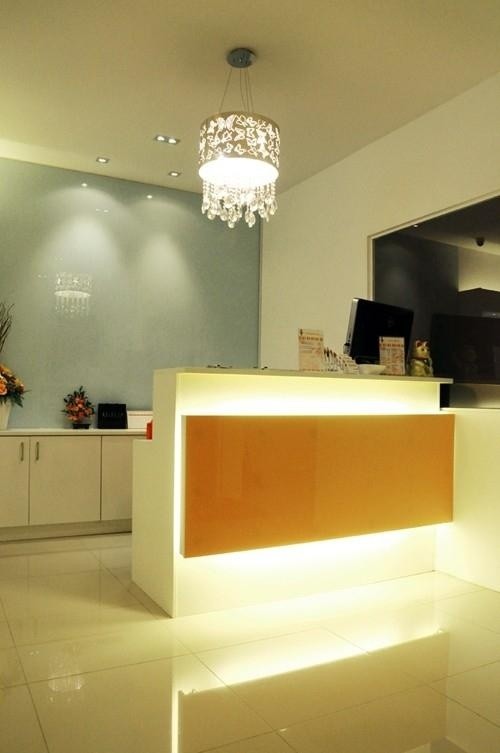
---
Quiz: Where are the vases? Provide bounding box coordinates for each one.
[72,422,92,428]
[0,398,13,430]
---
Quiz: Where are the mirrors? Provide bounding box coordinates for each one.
[365,191,500,409]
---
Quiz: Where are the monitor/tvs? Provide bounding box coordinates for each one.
[342,297,415,370]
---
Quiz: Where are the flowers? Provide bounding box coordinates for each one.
[0,301,26,408]
[60,385,98,423]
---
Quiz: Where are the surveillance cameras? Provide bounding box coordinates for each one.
[474,236,486,247]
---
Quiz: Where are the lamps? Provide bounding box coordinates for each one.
[53,270,94,318]
[197,47,282,230]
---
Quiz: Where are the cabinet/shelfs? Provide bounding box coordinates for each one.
[0,398,151,543]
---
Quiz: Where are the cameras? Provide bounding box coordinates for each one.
[431,311,499,384]
